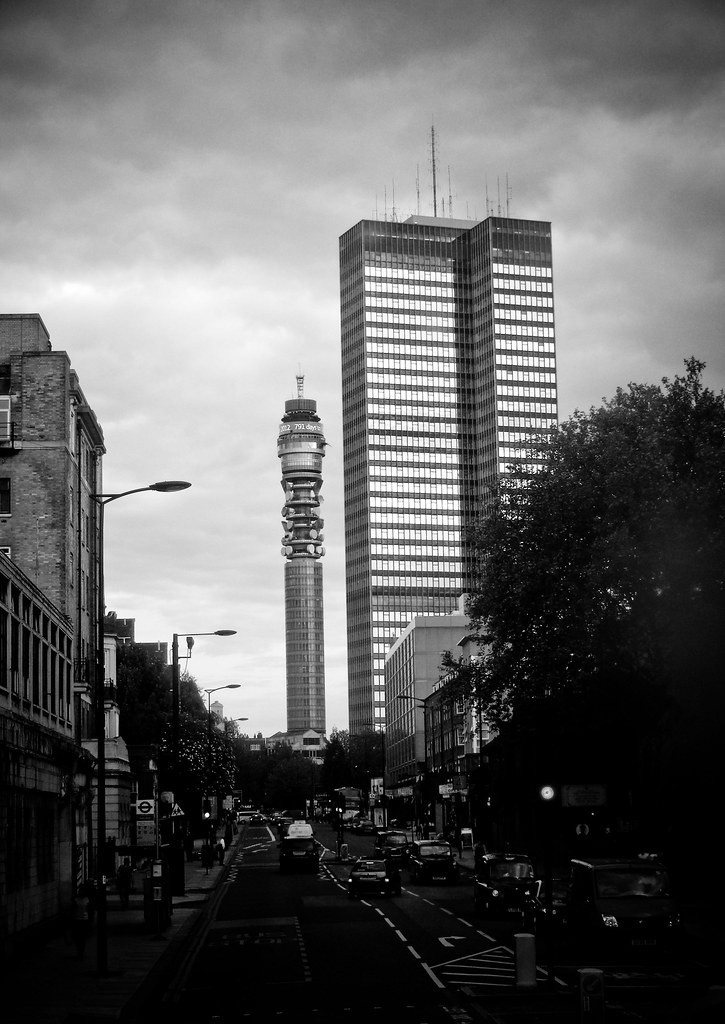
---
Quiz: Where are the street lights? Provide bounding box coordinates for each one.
[442,660,483,765]
[362,722,388,831]
[169,629,237,797]
[416,704,436,786]
[223,717,249,738]
[397,696,429,781]
[203,683,241,798]
[91,479,191,971]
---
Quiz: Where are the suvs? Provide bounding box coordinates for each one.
[519,854,687,965]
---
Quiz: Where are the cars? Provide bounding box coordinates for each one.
[406,837,458,886]
[336,853,404,900]
[237,806,375,849]
[469,849,540,923]
[372,828,411,865]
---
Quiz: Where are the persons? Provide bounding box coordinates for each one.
[114,858,135,902]
[455,836,466,859]
[222,808,237,833]
[473,839,487,871]
[311,802,332,824]
[413,823,430,840]
